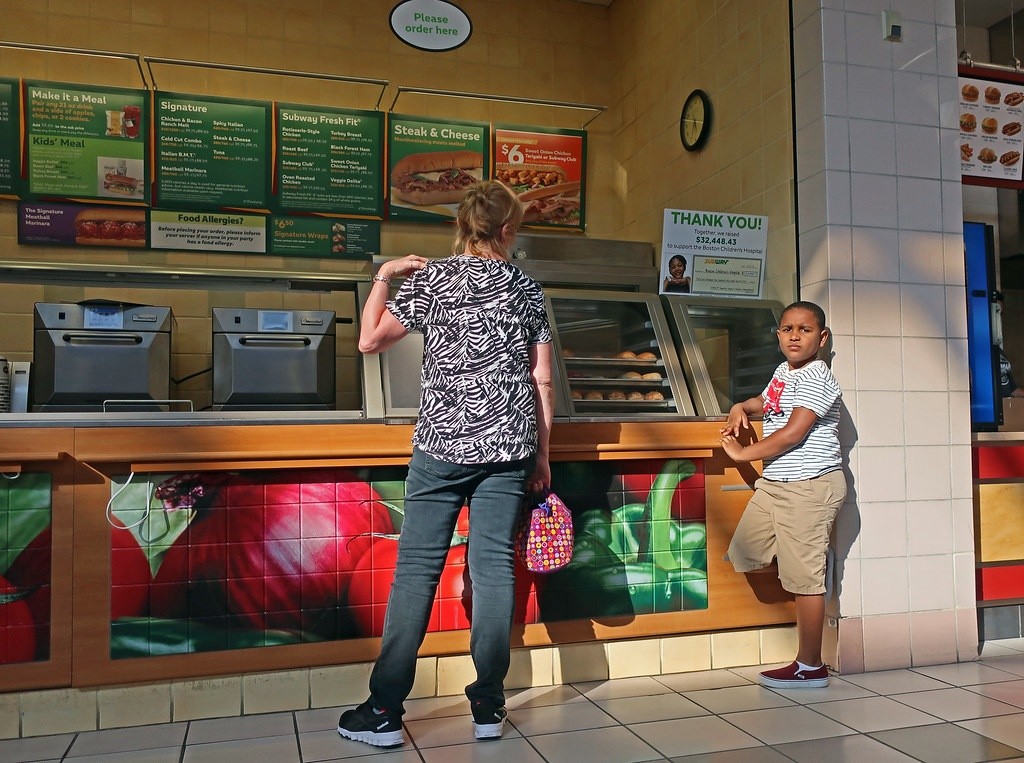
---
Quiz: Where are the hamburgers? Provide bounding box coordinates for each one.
[977,148,997,164]
[959,84,1001,133]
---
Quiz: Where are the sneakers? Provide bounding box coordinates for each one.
[759,660,829,689]
[472,702,508,739]
[824,547,834,601]
[337,700,405,746]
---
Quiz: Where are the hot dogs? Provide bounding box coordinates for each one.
[496,163,567,195]
[1004,92,1024,106]
[109,176,138,195]
[391,152,483,205]
[1002,122,1022,136]
[518,181,581,224]
[75,209,145,247]
[999,151,1021,166]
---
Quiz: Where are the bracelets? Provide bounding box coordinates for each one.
[373,275,391,285]
[665,276,672,282]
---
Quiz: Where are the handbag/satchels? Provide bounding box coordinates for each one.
[515,482,575,573]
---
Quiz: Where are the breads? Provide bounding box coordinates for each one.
[613,351,657,360]
[563,349,574,357]
[572,390,664,400]
[618,372,661,379]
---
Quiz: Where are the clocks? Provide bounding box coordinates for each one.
[679,89,712,151]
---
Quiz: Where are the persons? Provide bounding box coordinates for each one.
[718,301,847,688]
[336,180,554,747]
[662,255,691,293]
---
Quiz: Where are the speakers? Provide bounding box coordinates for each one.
[10,361,32,413]
